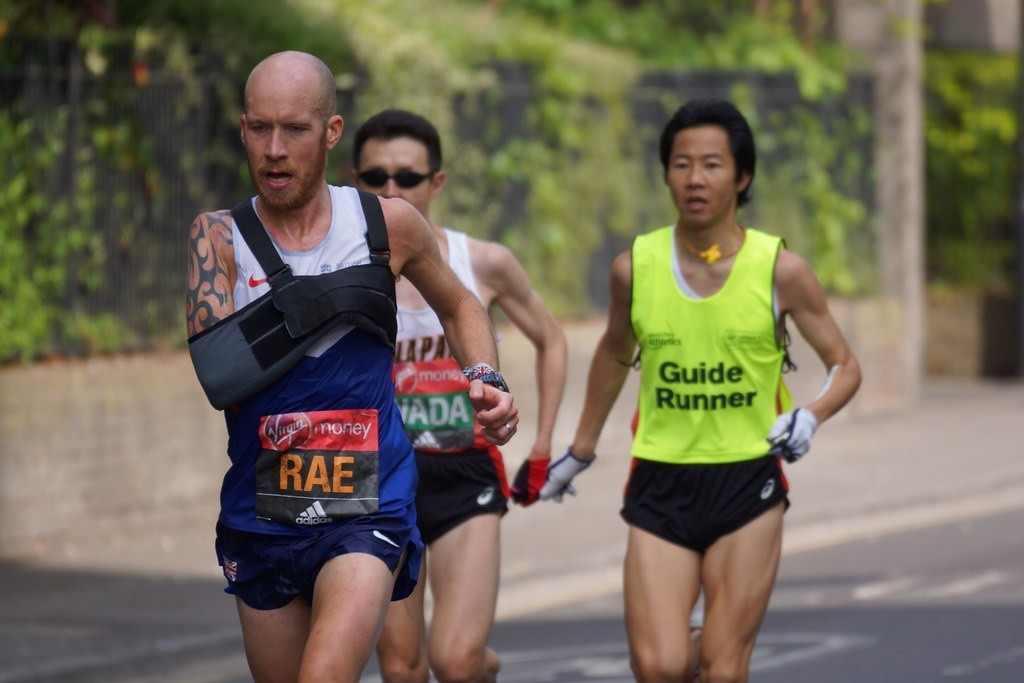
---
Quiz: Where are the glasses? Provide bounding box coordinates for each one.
[358,169,432,189]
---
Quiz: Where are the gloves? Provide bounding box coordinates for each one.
[538,447,597,503]
[509,458,550,507]
[765,407,818,464]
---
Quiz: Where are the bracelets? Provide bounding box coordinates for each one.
[463,363,493,379]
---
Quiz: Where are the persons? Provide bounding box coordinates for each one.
[186,50,520,683]
[539,99,861,683]
[352,109,568,682]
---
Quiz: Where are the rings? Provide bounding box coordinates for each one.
[504,423,512,432]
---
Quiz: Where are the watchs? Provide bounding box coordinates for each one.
[479,373,509,392]
